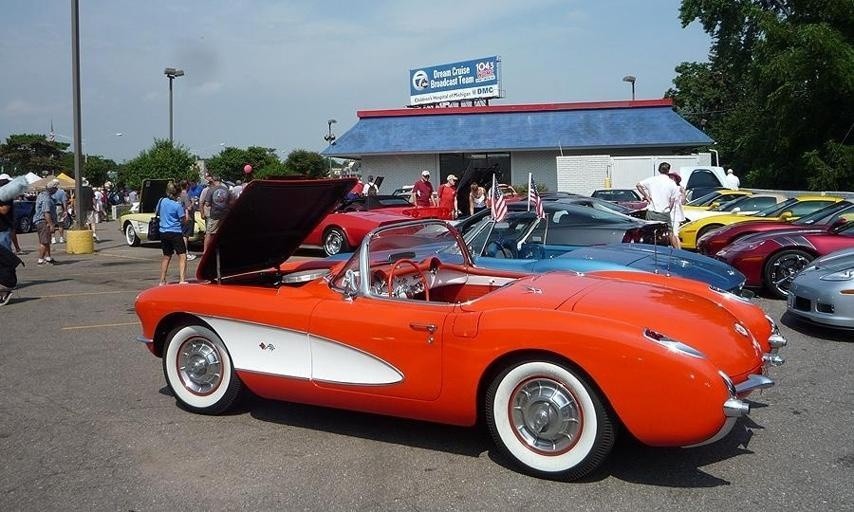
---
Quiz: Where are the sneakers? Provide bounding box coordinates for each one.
[37,258,53,267]
[51,237,56,244]
[179,282,189,284]
[93,233,96,241]
[0,292,14,306]
[15,250,27,254]
[46,256,60,265]
[159,281,167,287]
[59,237,64,243]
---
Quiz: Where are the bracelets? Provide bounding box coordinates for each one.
[454,210,458,212]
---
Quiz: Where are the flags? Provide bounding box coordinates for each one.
[487,178,507,222]
[530,174,545,221]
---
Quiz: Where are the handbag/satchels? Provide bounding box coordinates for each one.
[408,193,416,204]
[148,218,161,241]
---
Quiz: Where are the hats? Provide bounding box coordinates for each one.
[367,176,373,180]
[668,171,682,183]
[0,173,13,180]
[422,171,430,176]
[46,181,63,189]
[203,172,221,182]
[447,174,458,180]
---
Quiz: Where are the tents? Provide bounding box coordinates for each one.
[22,173,75,198]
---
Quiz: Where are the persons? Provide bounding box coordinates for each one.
[468,182,486,216]
[33,179,66,265]
[0,245,22,307]
[155,173,249,288]
[722,169,740,190]
[635,161,678,249]
[66,188,75,215]
[411,171,437,208]
[0,173,13,253]
[668,171,687,250]
[437,174,459,221]
[81,177,137,243]
[350,174,379,197]
[8,229,25,255]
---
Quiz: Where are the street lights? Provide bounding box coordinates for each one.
[164,67,184,152]
[46,131,126,165]
[324,118,337,176]
[623,74,636,100]
[170,142,227,161]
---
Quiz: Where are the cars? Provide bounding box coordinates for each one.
[11,199,72,232]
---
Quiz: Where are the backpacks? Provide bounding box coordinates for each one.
[368,183,376,198]
[210,186,230,220]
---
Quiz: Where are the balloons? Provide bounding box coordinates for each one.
[243,164,252,173]
